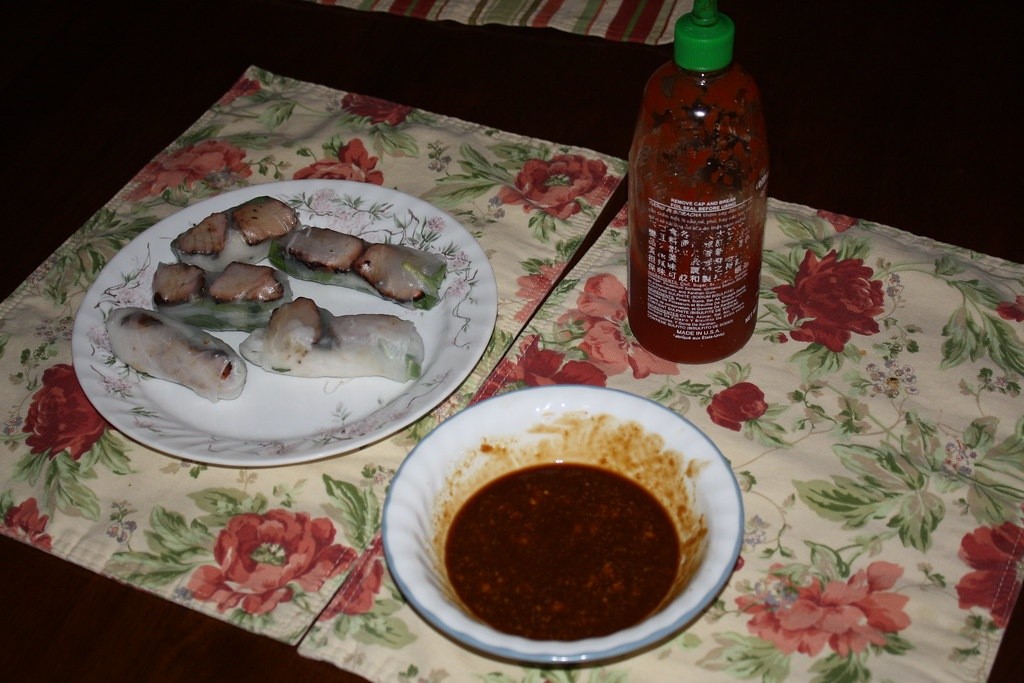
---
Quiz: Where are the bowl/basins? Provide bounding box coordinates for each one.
[381,383,744,666]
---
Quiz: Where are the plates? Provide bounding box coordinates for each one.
[71,178,499,468]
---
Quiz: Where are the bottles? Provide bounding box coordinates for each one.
[625,0,769,365]
